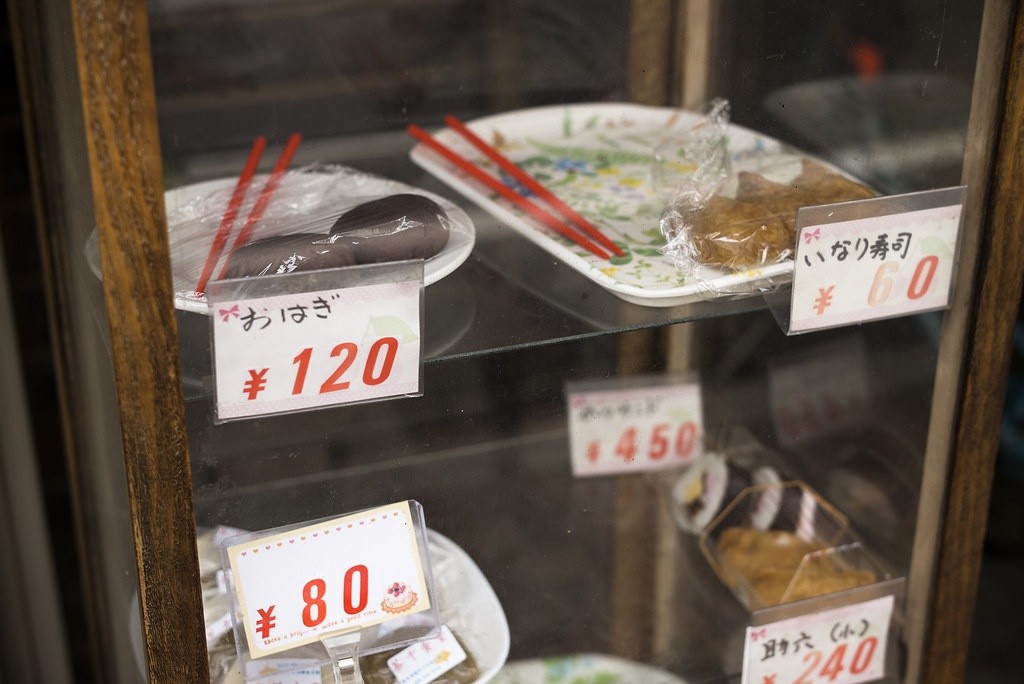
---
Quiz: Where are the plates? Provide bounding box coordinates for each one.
[131,522,511,684]
[85,172,477,315]
[488,651,689,684]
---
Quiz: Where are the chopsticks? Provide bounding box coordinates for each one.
[409,117,626,261]
[192,134,299,300]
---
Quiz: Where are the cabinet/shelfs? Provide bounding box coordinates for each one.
[71,97,945,684]
